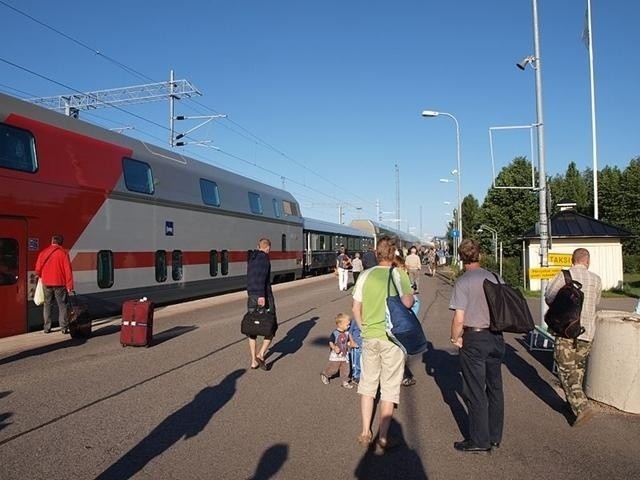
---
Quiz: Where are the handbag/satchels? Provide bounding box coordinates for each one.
[241,306,278,340]
[343,259,351,268]
[482,278,536,335]
[544,281,584,339]
[385,295,428,356]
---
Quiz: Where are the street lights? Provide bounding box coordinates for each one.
[440,178,461,266]
[418,106,463,268]
[338,205,364,226]
[476,223,498,262]
[444,200,458,251]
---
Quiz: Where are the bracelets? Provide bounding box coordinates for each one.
[450,338,457,345]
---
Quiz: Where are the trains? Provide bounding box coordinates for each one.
[0,91,447,342]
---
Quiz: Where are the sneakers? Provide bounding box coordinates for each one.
[572,406,597,428]
[402,377,417,386]
[252,353,272,371]
[320,373,360,390]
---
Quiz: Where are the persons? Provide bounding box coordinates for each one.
[448,239,507,454]
[544,249,602,426]
[35,234,76,334]
[336,246,450,294]
[348,319,362,385]
[351,236,413,456]
[392,255,420,387]
[320,313,353,389]
[246,238,278,371]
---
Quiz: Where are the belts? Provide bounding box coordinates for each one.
[462,326,491,333]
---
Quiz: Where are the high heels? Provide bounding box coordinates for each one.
[357,432,403,457]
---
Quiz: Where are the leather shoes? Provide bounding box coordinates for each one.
[454,439,500,452]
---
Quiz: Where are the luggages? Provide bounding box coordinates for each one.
[64,290,92,341]
[120,297,154,348]
